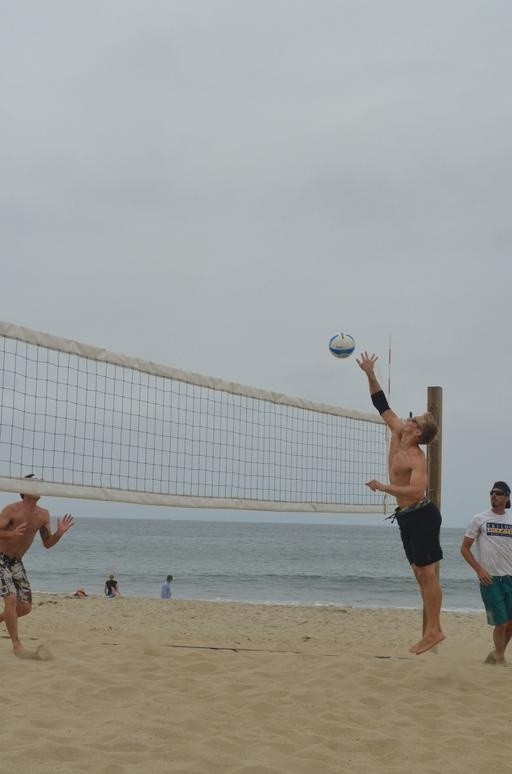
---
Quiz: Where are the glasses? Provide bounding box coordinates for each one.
[490,490,504,496]
[412,416,420,430]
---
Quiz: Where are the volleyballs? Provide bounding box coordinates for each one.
[329,333,355,358]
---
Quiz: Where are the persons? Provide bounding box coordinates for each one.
[161,575,173,599]
[0,474,74,654]
[356,350,445,656]
[104,575,118,598]
[461,480,512,662]
[75,586,88,600]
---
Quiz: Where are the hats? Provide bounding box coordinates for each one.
[493,481,510,508]
